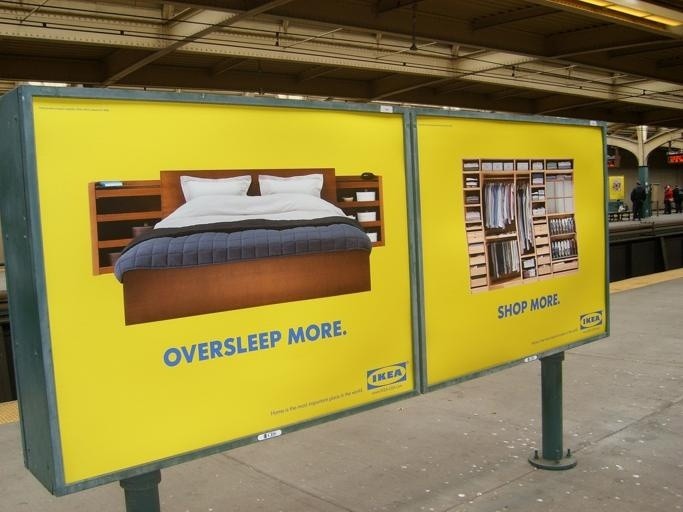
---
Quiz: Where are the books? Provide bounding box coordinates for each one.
[462,157,577,278]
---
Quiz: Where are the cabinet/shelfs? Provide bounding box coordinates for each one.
[335,174,385,247]
[461,157,580,292]
[86,178,163,275]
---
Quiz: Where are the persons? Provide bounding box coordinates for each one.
[617,199,625,212]
[664,184,673,214]
[630,181,647,221]
[672,184,682,213]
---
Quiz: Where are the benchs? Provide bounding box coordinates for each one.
[608,210,631,221]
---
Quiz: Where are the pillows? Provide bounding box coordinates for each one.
[257,173,324,198]
[179,175,253,202]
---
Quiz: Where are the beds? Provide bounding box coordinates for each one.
[114,168,373,325]
